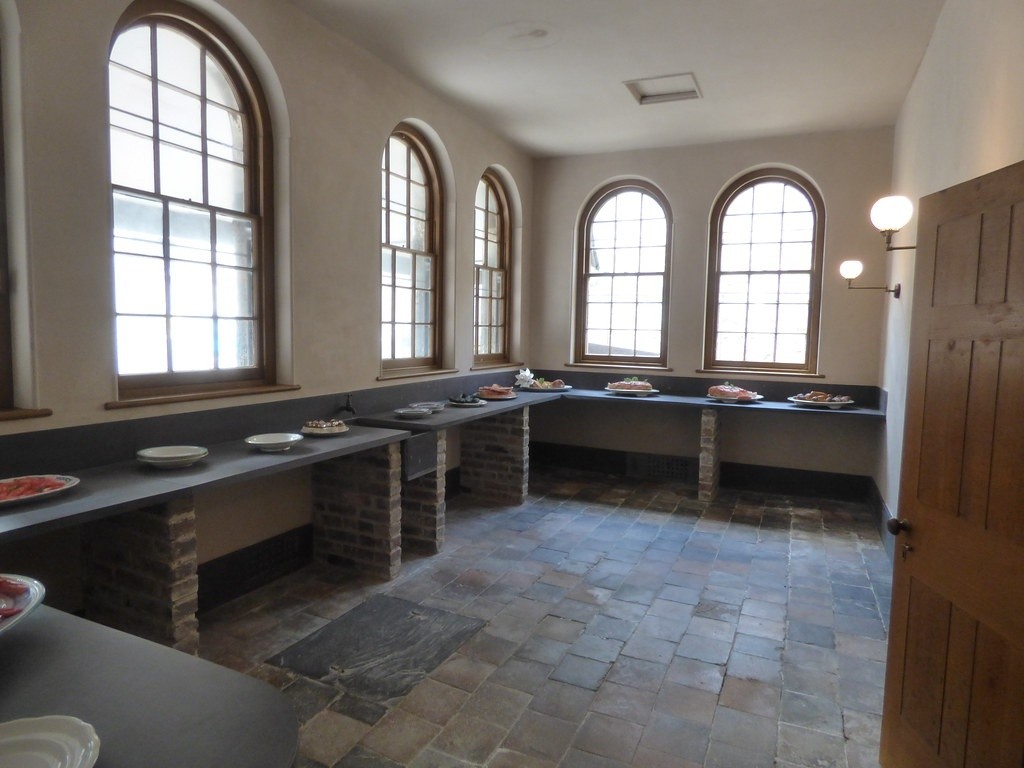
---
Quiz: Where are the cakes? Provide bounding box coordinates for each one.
[301,418,349,433]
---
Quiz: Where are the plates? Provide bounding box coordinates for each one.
[706,394,764,403]
[0,715,100,768]
[300,427,353,437]
[136,446,209,469]
[519,385,572,392]
[393,408,433,419]
[451,399,487,408]
[472,392,520,399]
[604,387,660,397]
[0,474,80,507]
[408,401,446,414]
[0,573,45,635]
[244,433,305,452]
[787,396,855,409]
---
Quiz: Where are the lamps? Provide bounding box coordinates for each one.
[870,195,916,251]
[840,260,900,298]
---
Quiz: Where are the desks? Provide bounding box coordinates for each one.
[0,386,885,768]
